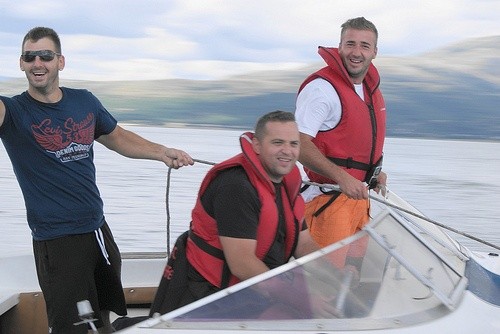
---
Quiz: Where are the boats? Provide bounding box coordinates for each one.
[0,179,500,333]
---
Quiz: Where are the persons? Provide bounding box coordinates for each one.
[0,25,194,334]
[147,109,361,323]
[292,15,388,319]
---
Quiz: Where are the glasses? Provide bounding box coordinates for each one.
[21,49,61,62]
